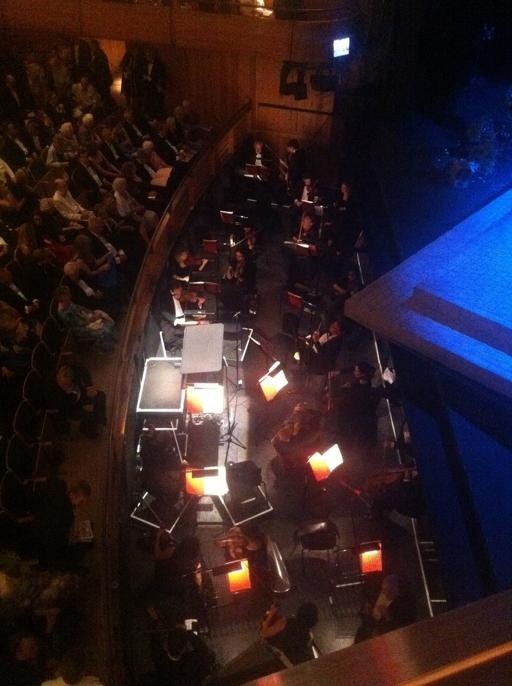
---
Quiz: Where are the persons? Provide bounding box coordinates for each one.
[0,35,412,686]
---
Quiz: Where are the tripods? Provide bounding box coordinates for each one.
[227,319,256,403]
[219,367,248,449]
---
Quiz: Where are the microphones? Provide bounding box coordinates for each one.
[223,356,228,367]
[233,312,240,318]
